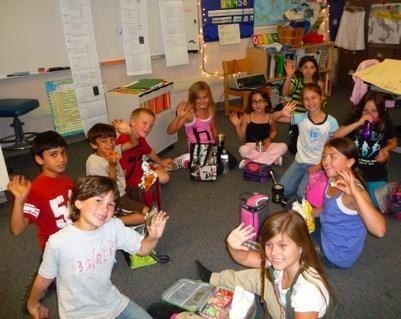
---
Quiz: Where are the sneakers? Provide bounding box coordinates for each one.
[164,162,179,172]
[239,159,246,169]
[274,156,283,166]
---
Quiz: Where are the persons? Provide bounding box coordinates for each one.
[228,89,290,170]
[334,92,398,213]
[86,118,156,222]
[30,176,170,318]
[279,55,331,157]
[9,130,151,311]
[268,83,342,199]
[146,213,338,318]
[281,134,389,273]
[118,107,191,206]
[167,81,239,175]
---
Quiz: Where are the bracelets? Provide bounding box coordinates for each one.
[266,135,273,142]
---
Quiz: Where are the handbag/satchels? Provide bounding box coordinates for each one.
[189,143,218,181]
[129,203,169,270]
[240,191,271,241]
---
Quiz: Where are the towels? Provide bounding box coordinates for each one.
[335,10,366,51]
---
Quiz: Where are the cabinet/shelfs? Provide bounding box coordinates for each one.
[104,79,178,163]
[247,31,335,96]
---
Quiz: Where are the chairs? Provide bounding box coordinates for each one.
[223,58,271,118]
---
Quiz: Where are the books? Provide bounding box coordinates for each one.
[114,78,165,92]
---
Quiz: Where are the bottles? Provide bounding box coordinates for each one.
[221,148,231,175]
[361,114,372,139]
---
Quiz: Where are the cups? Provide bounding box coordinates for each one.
[255,140,266,152]
[271,184,284,202]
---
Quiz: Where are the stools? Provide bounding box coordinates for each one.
[0,100,42,174]
